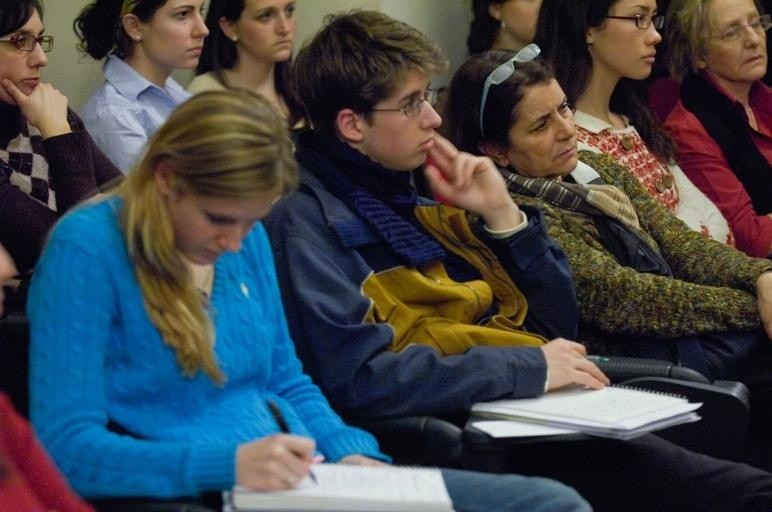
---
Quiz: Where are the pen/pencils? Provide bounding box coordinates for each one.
[266,399,316,482]
[587,355,609,363]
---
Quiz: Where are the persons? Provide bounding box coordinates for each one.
[1,2,772,512]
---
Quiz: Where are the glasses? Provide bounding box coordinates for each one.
[372,89,438,118]
[721,14,772,42]
[480,43,541,137]
[0,33,53,52]
[602,14,664,29]
